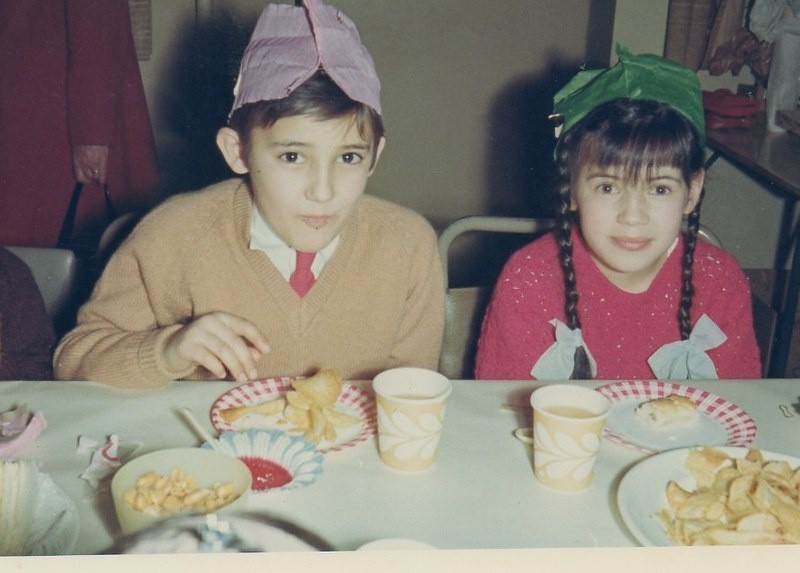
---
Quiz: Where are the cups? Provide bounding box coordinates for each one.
[530,383,612,488]
[108,448,253,536]
[371,366,452,472]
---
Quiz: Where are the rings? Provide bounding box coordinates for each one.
[93,168,99,173]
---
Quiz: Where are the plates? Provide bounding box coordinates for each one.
[596,380,756,453]
[211,375,378,455]
[616,446,800,545]
[0,475,78,556]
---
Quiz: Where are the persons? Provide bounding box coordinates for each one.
[48,0,445,379]
[0,0,161,381]
[478,44,761,379]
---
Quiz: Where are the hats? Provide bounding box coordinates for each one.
[227,1,382,126]
[553,43,706,153]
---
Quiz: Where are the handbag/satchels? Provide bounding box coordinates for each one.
[48,177,117,338]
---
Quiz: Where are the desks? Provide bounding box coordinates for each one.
[703,111,800,379]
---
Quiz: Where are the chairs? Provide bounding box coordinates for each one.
[437,215,724,380]
[6,245,78,331]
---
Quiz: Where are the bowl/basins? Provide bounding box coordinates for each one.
[201,430,324,495]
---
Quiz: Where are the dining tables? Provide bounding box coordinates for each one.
[0,378,800,556]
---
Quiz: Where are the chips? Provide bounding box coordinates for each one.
[285,366,341,405]
[655,445,800,546]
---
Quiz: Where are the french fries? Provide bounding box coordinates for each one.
[220,398,358,442]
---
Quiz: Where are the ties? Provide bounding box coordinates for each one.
[289,251,316,297]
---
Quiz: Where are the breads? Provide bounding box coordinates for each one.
[637,396,697,425]
